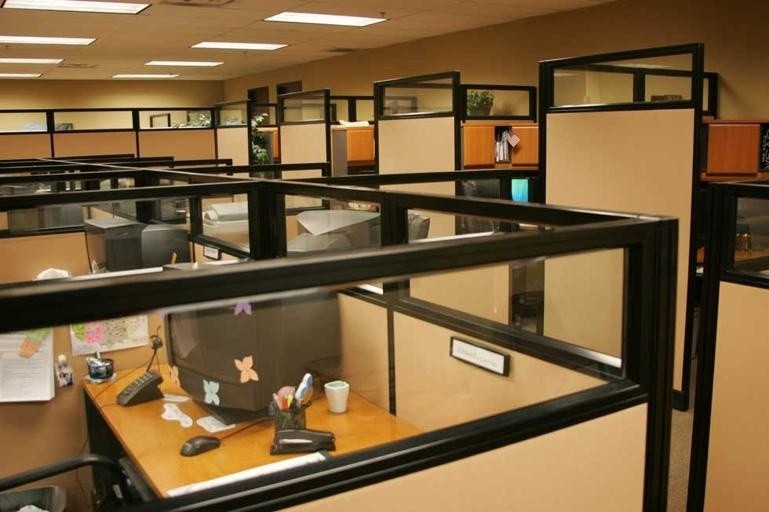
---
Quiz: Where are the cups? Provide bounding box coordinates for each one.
[323,380,350,414]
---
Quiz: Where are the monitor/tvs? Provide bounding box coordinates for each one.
[84,217,190,275]
[8,204,83,230]
[163,262,341,424]
[510,176,531,203]
[287,210,381,254]
[205,201,249,225]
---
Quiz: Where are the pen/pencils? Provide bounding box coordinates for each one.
[271,384,314,430]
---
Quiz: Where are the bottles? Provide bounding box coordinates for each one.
[741,234,752,257]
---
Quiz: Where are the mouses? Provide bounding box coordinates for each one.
[180,437,220,455]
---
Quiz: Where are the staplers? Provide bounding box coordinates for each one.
[269,429,336,454]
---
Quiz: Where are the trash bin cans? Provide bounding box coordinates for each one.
[512,289,543,316]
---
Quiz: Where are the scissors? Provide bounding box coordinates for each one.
[295,373,315,406]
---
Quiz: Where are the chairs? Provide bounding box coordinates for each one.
[0,454,130,512]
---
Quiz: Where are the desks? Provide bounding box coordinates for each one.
[80,359,424,512]
